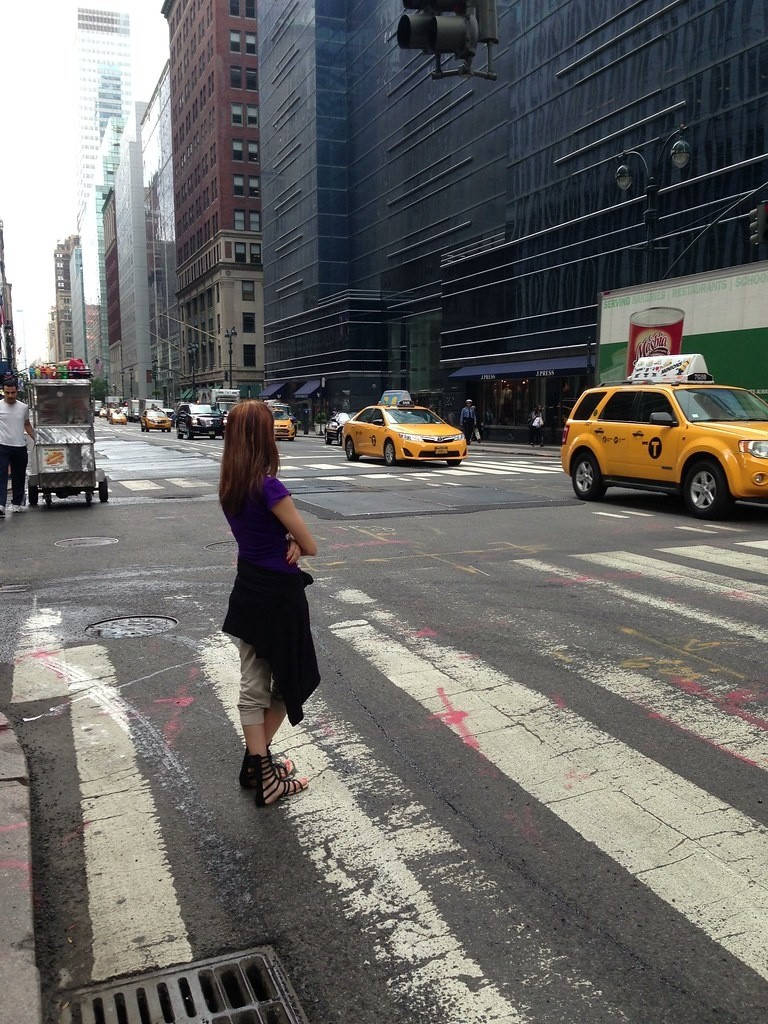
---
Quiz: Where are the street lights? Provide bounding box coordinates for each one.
[613,124,695,284]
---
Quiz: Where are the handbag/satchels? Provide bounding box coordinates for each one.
[531,413,544,428]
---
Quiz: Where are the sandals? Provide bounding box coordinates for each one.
[239,738,294,791]
[248,748,309,808]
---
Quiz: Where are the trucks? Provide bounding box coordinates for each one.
[138,399,176,425]
[128,398,141,423]
[197,389,241,434]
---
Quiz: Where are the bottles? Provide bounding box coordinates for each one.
[30,365,90,379]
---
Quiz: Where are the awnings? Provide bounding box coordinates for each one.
[292,380,325,399]
[181,385,222,402]
[447,355,595,383]
[256,383,288,398]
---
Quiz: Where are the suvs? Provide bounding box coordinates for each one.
[176,401,227,440]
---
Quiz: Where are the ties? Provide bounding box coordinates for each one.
[467,408,471,420]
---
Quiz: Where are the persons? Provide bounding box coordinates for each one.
[470,405,482,443]
[527,402,544,447]
[460,399,476,444]
[502,384,513,422]
[0,378,35,517]
[217,399,322,808]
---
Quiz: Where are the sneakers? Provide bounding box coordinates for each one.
[12,504,22,513]
[0,505,7,515]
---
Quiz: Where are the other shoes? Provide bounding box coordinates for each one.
[478,439,480,443]
[466,441,469,445]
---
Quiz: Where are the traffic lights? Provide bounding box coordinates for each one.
[748,200,768,246]
[396,1,499,81]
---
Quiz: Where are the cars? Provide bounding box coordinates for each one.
[342,389,469,467]
[324,412,359,446]
[265,406,296,442]
[560,354,768,520]
[95,400,128,425]
[140,404,172,432]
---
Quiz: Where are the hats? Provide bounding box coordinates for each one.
[465,399,472,403]
